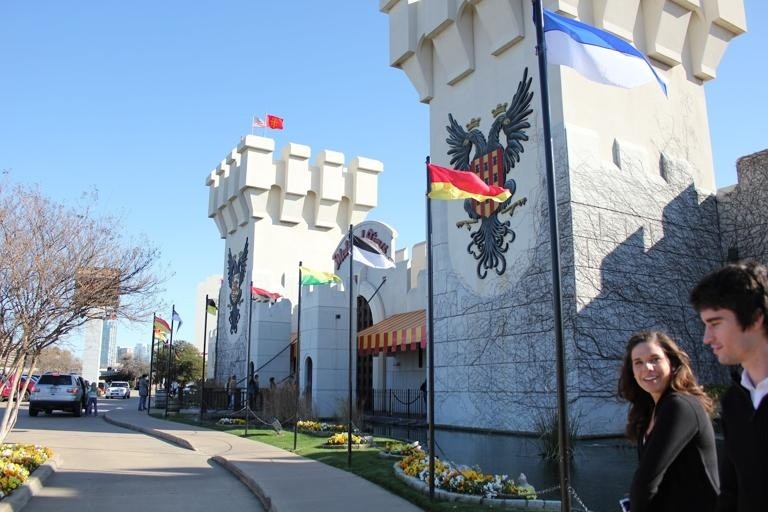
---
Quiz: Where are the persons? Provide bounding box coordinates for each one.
[687,258,767,512]
[613,330,721,511]
[253,374,260,393]
[85,382,100,417]
[137,373,148,411]
[419,378,428,407]
[224,376,233,410]
[227,374,239,411]
[268,377,276,390]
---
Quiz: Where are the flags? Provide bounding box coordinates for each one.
[300,266,343,286]
[424,163,513,205]
[252,114,265,129]
[530,1,671,104]
[153,315,171,335]
[266,114,284,131]
[249,286,283,309]
[348,233,397,269]
[153,326,167,342]
[206,299,218,316]
[171,310,183,332]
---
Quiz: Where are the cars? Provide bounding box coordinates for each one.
[170,381,179,393]
[0,373,132,417]
[182,381,198,394]
